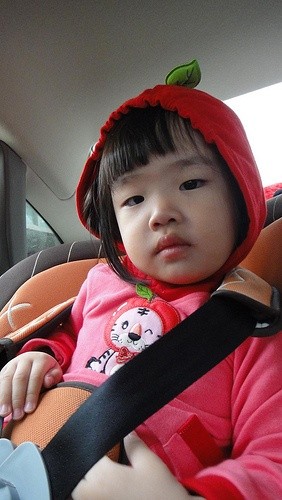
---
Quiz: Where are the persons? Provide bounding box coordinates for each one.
[0,89,282,500]
